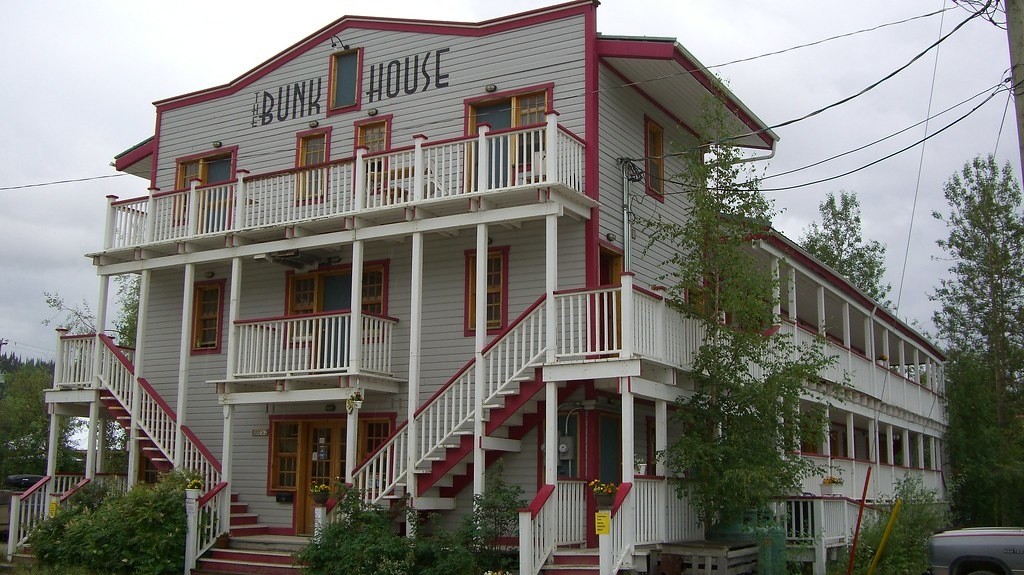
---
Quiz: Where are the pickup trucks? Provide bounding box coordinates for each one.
[928,528,1024,575]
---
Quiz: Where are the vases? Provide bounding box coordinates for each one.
[312,492,330,503]
[352,401,363,409]
[637,463,647,476]
[185,488,200,499]
[876,359,883,366]
[820,483,844,497]
[597,493,615,506]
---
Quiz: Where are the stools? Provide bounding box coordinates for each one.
[378,187,408,206]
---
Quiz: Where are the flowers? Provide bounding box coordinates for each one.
[350,391,363,402]
[823,474,844,485]
[588,478,620,495]
[878,355,888,362]
[184,468,206,489]
[309,479,331,493]
[634,452,644,474]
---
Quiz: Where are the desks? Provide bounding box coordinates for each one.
[366,164,432,179]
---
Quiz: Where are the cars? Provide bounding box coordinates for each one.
[1,475,45,530]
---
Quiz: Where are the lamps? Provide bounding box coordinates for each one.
[212,141,223,148]
[485,84,496,93]
[308,120,318,128]
[205,271,215,278]
[606,233,618,242]
[368,108,378,116]
[487,237,493,245]
[324,404,336,411]
